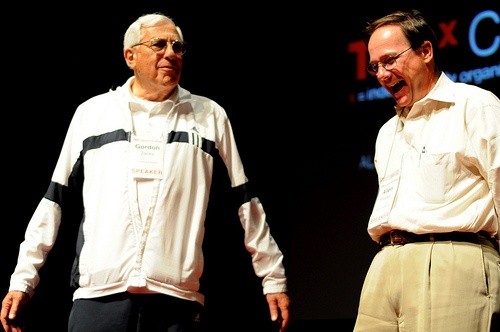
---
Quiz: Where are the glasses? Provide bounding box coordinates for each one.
[131,37,189,56]
[367,47,413,76]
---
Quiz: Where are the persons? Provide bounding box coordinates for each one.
[0,14,289,332]
[352,12,500,332]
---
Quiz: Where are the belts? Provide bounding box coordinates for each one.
[379,229,499,253]
